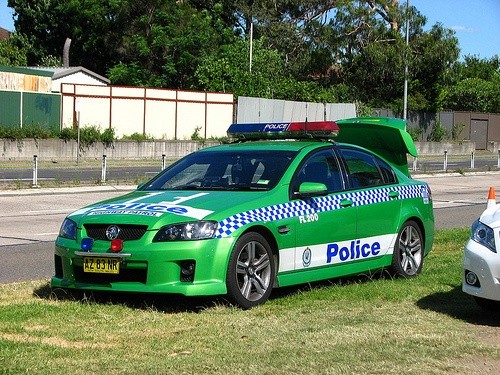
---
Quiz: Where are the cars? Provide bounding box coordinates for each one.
[462,203,500,302]
[51,116,434,310]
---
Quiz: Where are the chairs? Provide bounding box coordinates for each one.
[294,162,337,192]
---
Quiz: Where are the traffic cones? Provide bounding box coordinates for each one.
[486,186,497,208]
